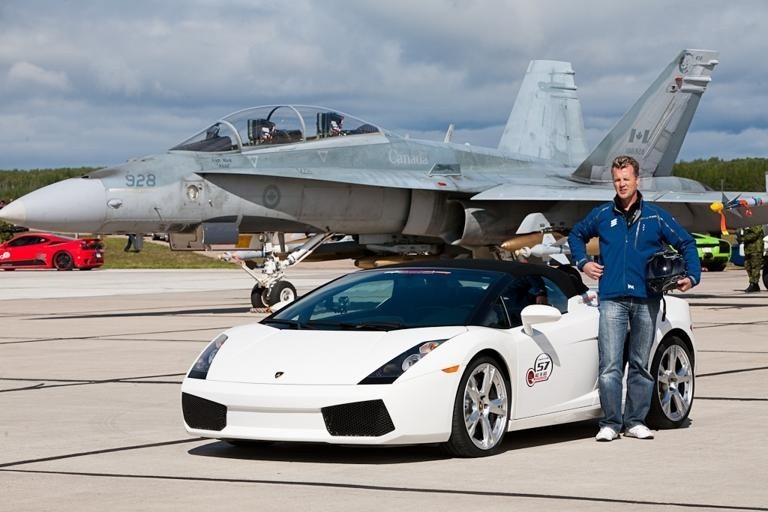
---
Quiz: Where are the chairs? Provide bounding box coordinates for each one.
[268,123,371,143]
[378,285,532,327]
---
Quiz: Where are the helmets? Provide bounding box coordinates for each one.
[646,248,685,293]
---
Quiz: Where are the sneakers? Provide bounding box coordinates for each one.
[595,426,619,441]
[744,282,760,292]
[624,424,654,439]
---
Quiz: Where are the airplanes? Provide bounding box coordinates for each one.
[0,48,768,311]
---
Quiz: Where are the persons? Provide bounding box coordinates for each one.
[564,156,703,444]
[733,224,765,295]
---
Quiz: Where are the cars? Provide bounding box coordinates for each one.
[739,235,768,289]
[0,232,103,271]
[669,233,732,271]
[180,258,697,458]
